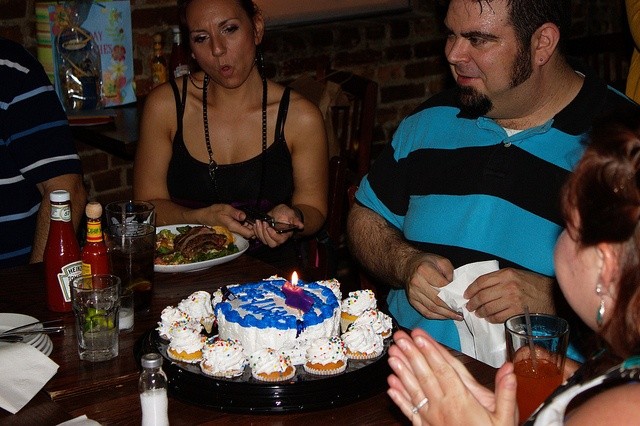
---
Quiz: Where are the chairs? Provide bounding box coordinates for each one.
[317,59,378,182]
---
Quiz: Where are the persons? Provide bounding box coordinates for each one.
[347,0,640,367]
[1,40,87,266]
[387,117,639,426]
[133,0,329,247]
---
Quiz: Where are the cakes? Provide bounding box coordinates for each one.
[153,273,395,386]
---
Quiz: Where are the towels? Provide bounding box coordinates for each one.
[430,259,507,369]
[0,340,60,414]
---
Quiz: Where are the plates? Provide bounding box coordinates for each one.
[156,225,250,273]
[3,312,53,358]
[149,318,394,386]
[1,309,54,367]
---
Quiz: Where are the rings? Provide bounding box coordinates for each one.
[413,398,428,413]
[412,396,427,413]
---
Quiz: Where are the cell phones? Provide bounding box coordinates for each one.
[227,204,301,236]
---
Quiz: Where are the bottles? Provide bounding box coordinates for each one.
[137,353,169,426]
[43,188,82,312]
[80,201,111,287]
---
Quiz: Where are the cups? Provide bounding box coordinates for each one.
[101,283,136,336]
[69,273,120,363]
[505,313,570,423]
[105,199,155,303]
